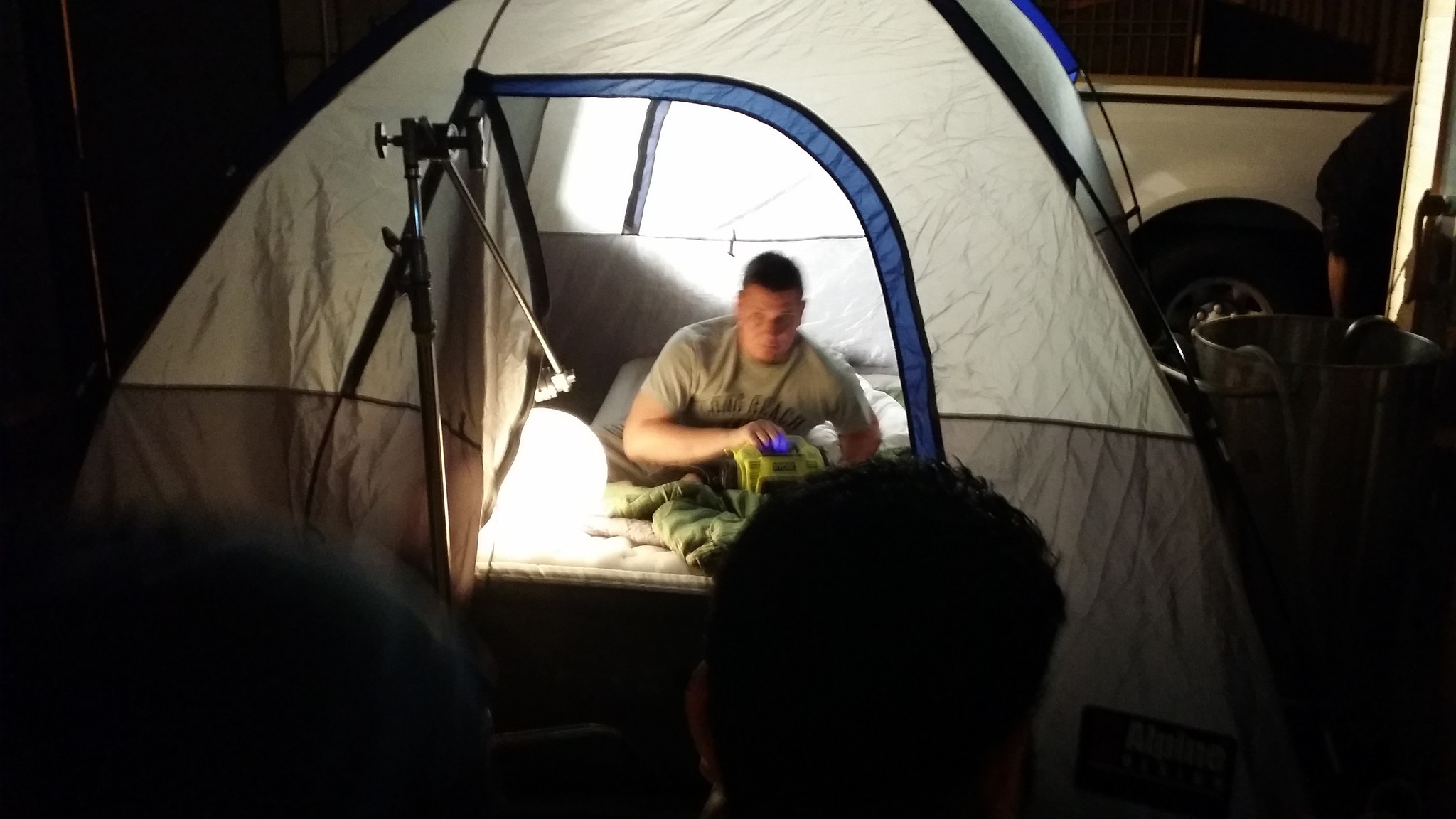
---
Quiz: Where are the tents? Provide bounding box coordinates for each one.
[68,3,1319,819]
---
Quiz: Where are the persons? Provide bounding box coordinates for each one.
[688,453,1066,819]
[621,250,883,470]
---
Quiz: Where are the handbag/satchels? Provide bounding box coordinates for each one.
[735,435,826,495]
